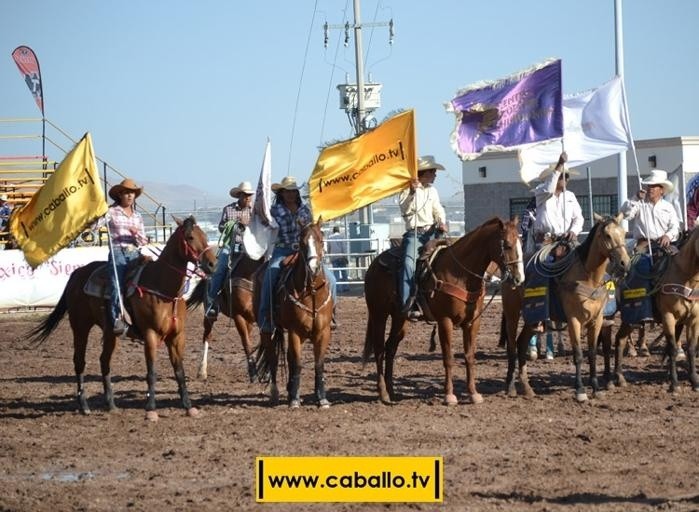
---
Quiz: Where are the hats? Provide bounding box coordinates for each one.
[271,176,302,194]
[641,170,674,194]
[418,156,444,171]
[230,183,255,197]
[109,179,142,200]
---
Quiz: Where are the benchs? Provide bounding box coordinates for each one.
[0,161,56,244]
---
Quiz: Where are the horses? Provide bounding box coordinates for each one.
[360,214,525,406]
[185,221,265,384]
[20,215,218,422]
[499,212,630,402]
[242,215,335,410]
[601,225,699,392]
[529,317,566,360]
[626,325,686,361]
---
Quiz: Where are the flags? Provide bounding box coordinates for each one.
[257,143,282,229]
[8,133,109,269]
[444,58,563,162]
[308,108,417,224]
[518,77,630,184]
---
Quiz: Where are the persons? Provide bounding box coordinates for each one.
[0,197,10,231]
[520,196,536,238]
[91,178,146,336]
[686,186,699,231]
[535,151,584,244]
[398,156,449,322]
[620,170,680,253]
[206,181,256,321]
[257,177,337,334]
